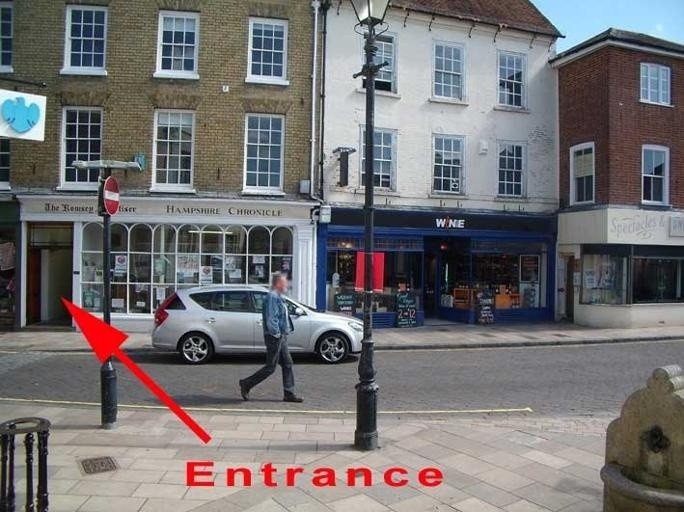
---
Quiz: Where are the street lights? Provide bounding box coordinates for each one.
[337,1,390,453]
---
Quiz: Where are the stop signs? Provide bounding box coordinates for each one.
[103,177,122,216]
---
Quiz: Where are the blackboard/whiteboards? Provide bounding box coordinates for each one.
[475,290,496,325]
[334,293,356,316]
[396,291,419,328]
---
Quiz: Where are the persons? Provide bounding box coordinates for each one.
[6,276,17,313]
[130,273,141,294]
[239,274,306,403]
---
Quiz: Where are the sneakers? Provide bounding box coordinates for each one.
[239,378,248,400]
[283,396,303,403]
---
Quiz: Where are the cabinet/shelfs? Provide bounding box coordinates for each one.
[453,288,520,309]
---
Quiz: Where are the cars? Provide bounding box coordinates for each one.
[150,283,363,366]
